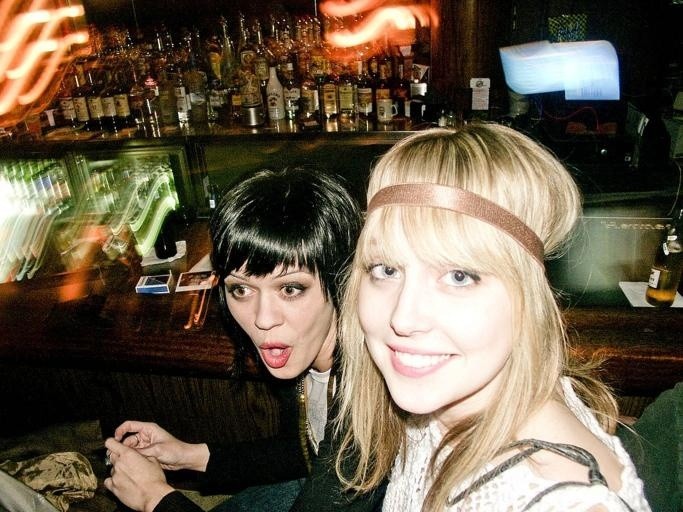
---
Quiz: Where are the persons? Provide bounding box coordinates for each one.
[332,122,651,512]
[105,161,389,512]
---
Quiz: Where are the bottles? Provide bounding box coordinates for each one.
[646,210,683,307]
[0,12,430,138]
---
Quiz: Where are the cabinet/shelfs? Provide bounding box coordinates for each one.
[2,117,426,224]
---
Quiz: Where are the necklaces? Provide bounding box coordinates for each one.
[296,373,312,472]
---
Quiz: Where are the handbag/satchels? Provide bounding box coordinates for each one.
[0,452,99,512]
